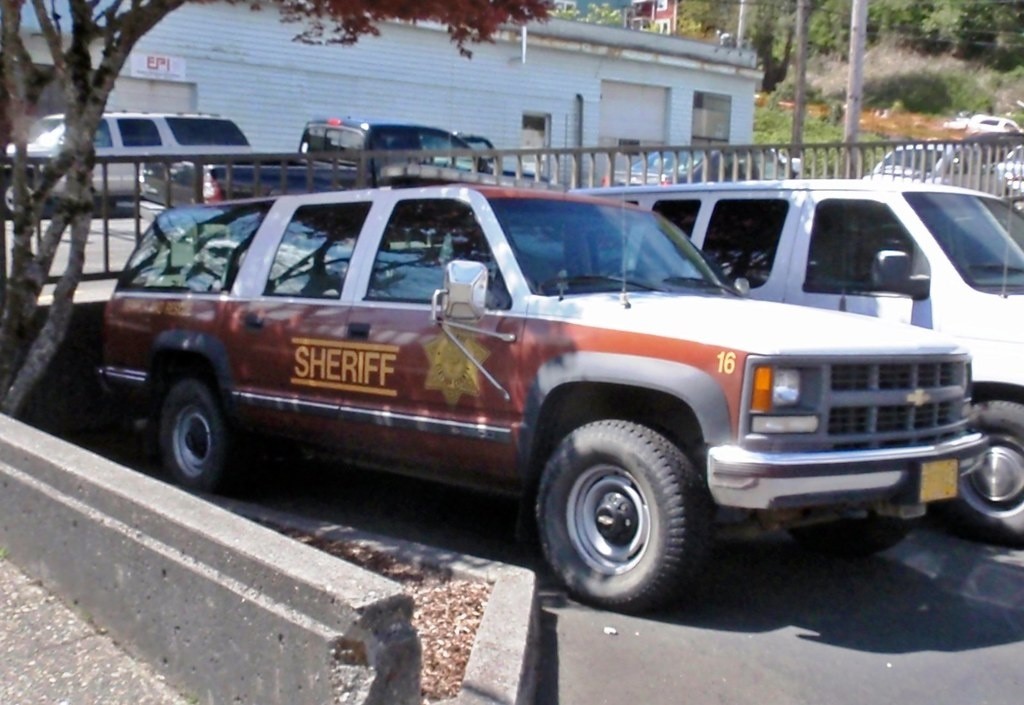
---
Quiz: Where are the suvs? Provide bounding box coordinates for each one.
[94,179,988,610]
[565,173,1024,549]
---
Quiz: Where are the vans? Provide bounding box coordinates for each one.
[1,114,257,222]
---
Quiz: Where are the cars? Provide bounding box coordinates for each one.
[599,149,803,200]
[859,143,1008,200]
[950,113,1024,216]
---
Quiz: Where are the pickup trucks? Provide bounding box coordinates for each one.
[140,116,553,231]
